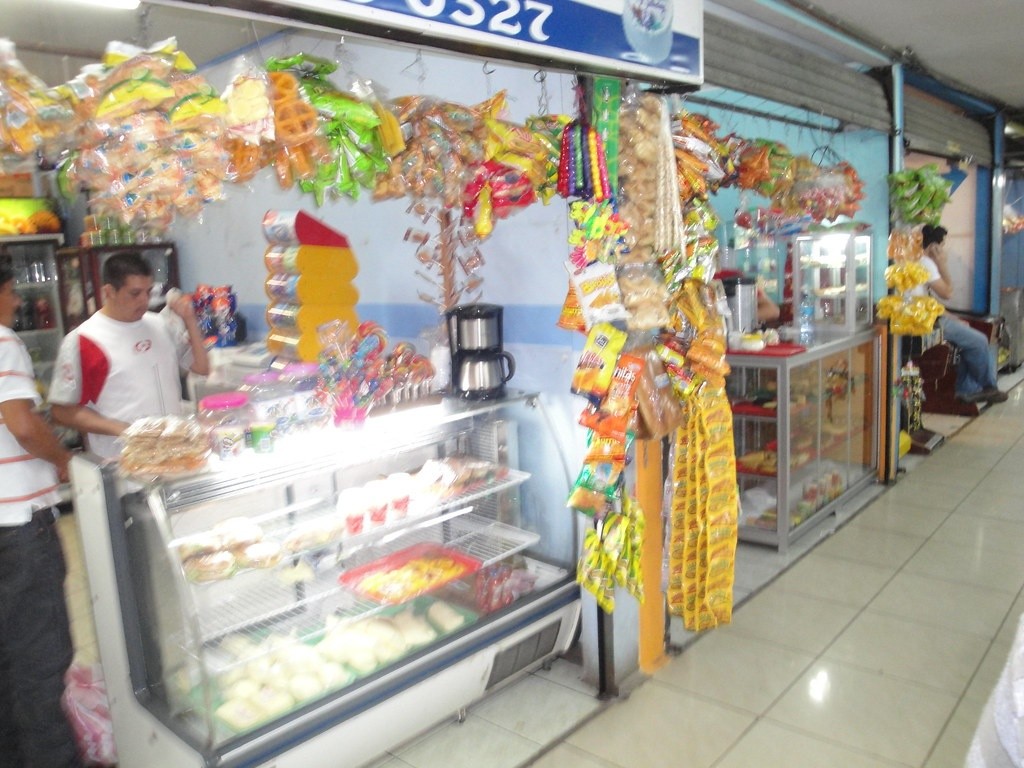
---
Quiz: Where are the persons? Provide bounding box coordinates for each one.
[48,253,212,460]
[912,223,1009,403]
[0,248,99,768]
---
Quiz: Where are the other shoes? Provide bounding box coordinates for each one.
[956,388,1008,404]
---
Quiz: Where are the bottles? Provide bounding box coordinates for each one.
[193,389,254,460]
[236,370,296,447]
[14,291,54,334]
[281,364,327,433]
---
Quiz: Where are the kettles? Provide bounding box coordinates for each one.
[447,305,514,401]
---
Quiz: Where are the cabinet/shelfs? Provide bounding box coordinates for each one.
[0,237,87,509]
[792,229,873,335]
[69,380,584,768]
[708,325,878,555]
